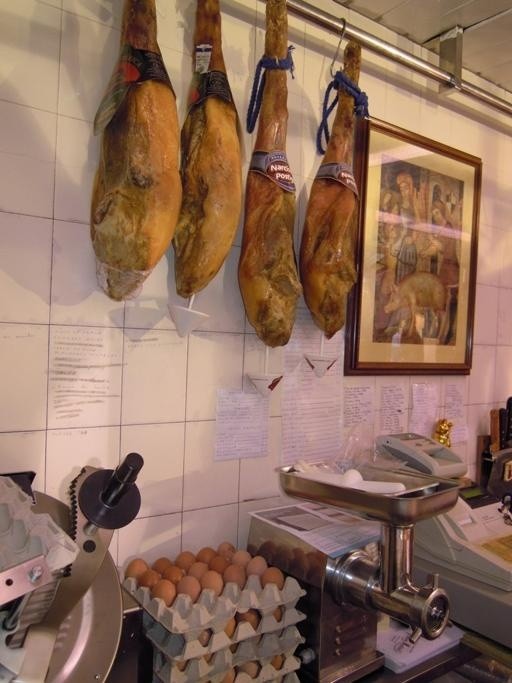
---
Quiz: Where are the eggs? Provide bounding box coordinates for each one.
[125,542,285,683]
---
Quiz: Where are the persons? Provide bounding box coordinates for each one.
[382,170,459,349]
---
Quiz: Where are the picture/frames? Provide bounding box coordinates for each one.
[343,110,483,379]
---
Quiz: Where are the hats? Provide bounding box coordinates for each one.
[396,170,415,199]
[433,200,447,216]
